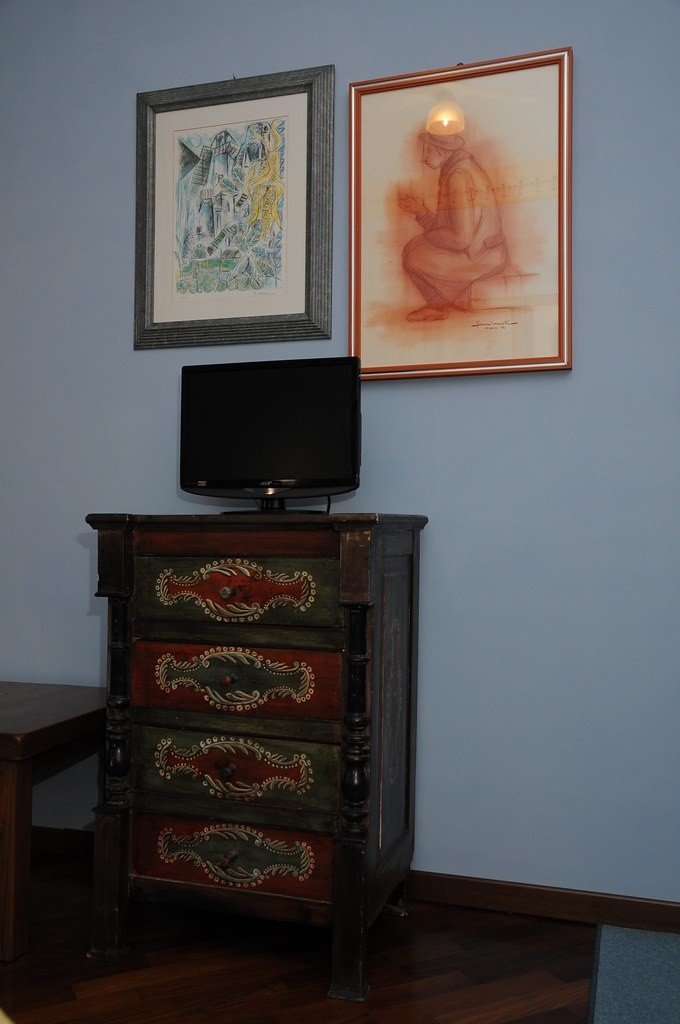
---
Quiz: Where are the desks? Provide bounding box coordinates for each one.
[0,678,108,964]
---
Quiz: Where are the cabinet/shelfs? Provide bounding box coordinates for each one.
[85,511,428,1003]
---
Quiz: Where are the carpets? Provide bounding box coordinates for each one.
[586,923,680,1024]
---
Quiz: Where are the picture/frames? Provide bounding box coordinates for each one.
[130,63,337,351]
[348,46,575,382]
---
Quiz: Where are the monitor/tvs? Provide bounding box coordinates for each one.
[179,357,361,518]
